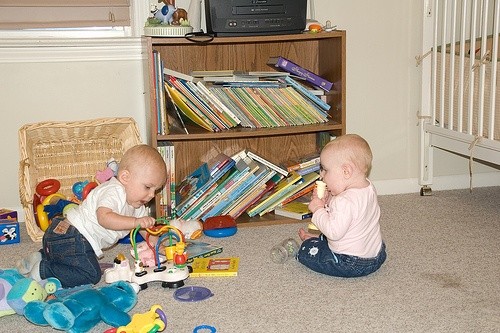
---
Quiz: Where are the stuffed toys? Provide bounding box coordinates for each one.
[0,269,138,333]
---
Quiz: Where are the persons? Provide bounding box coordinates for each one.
[295,134,387,278]
[18,144,167,289]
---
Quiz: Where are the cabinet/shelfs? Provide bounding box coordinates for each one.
[151,30,346,227]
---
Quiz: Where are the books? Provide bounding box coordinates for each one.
[158,141,321,224]
[190,257,239,277]
[152,49,333,135]
[183,241,223,264]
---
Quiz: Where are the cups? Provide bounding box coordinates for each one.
[270,238,301,264]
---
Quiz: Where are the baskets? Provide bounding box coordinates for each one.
[18,117,142,242]
